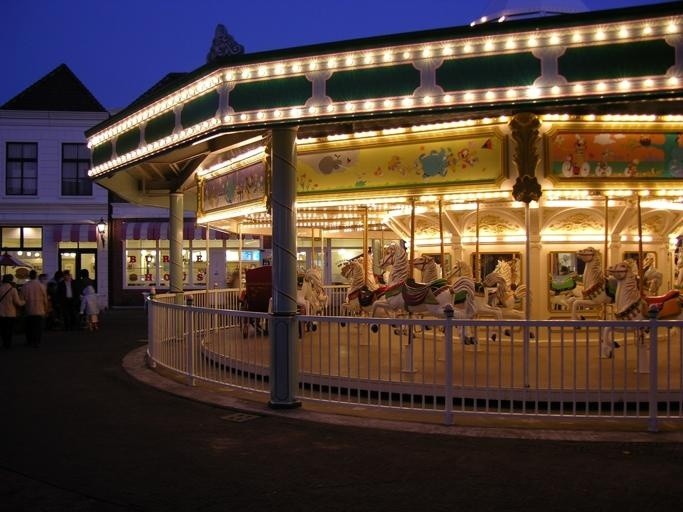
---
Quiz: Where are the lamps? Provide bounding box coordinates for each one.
[97,216,106,247]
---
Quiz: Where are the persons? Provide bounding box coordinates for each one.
[0,267,99,346]
